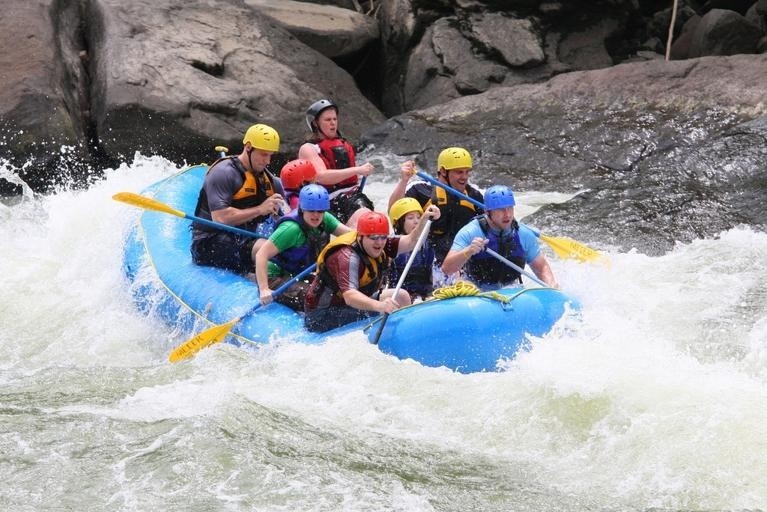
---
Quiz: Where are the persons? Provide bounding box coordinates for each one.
[390,147,484,269]
[251,160,317,264]
[303,205,442,334]
[441,184,552,287]
[297,98,375,224]
[256,185,352,312]
[380,198,435,306]
[191,124,282,271]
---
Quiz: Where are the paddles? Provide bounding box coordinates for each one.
[113,193,272,241]
[168,262,318,364]
[409,167,599,262]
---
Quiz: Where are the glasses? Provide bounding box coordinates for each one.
[367,234,387,240]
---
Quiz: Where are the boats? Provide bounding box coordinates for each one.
[118,164,583,375]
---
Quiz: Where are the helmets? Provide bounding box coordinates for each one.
[305,98,339,132]
[242,123,280,154]
[280,158,317,192]
[436,146,474,173]
[388,197,424,225]
[356,211,391,238]
[482,184,517,211]
[298,183,331,212]
